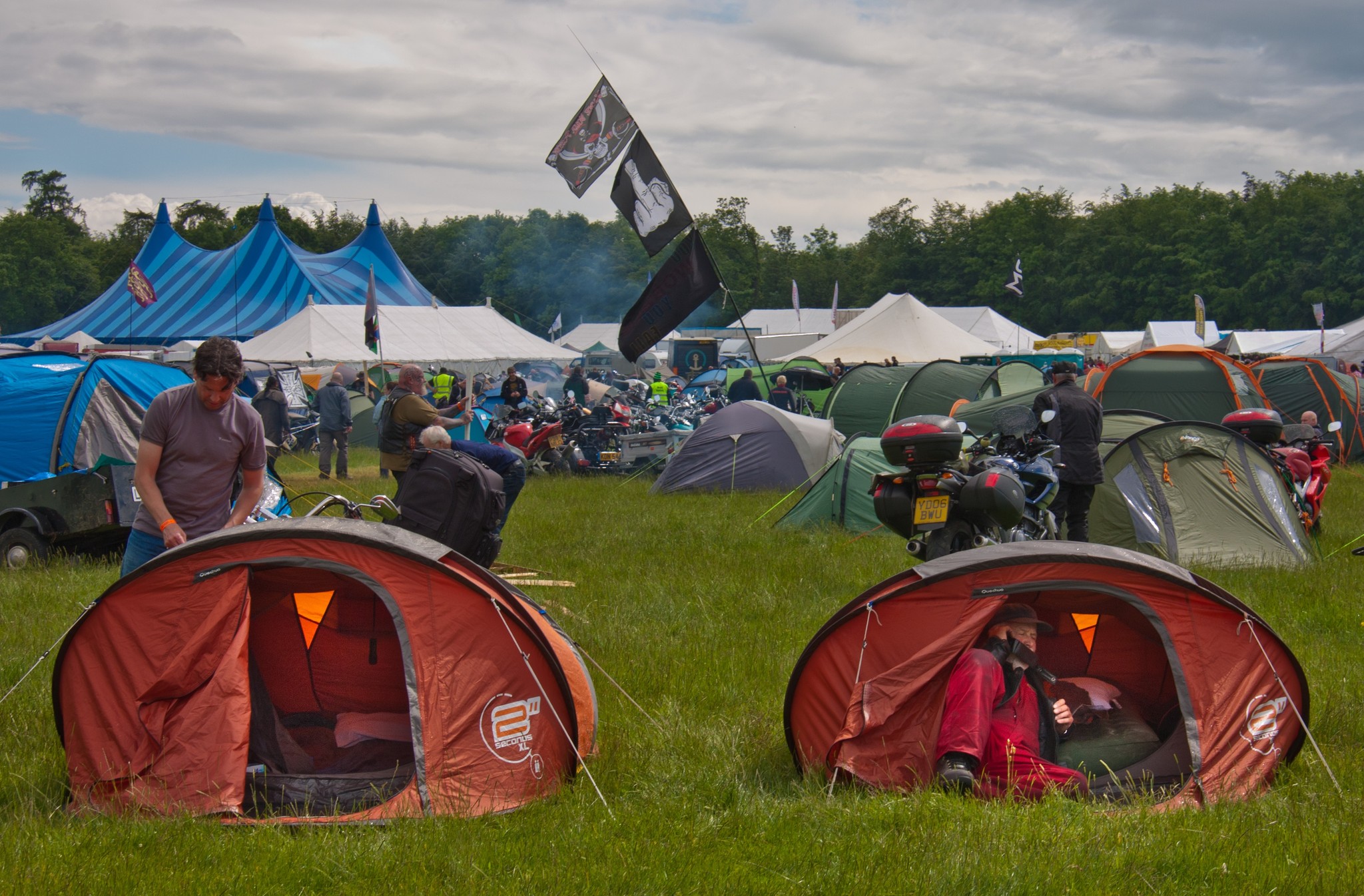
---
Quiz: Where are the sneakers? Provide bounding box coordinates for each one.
[938,753,973,796]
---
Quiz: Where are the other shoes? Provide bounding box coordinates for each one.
[337,475,354,480]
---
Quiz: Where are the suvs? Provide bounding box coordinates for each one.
[512,360,563,381]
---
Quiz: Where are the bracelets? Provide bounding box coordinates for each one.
[457,402,463,411]
[160,519,176,532]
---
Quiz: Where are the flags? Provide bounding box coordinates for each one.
[364,270,376,349]
[545,76,721,365]
[369,315,380,355]
[1002,255,1024,298]
[127,260,158,308]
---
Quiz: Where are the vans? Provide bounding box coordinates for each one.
[569,349,669,375]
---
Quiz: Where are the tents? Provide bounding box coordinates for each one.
[782,540,1310,813]
[51,517,598,828]
[0,194,1364,575]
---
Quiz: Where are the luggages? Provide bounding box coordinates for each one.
[381,449,507,569]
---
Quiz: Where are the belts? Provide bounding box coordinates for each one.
[510,459,522,469]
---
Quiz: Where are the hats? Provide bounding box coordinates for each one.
[653,371,662,380]
[987,603,1053,633]
[1053,362,1077,374]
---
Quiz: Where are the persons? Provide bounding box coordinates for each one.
[1301,411,1324,440]
[426,367,459,406]
[1024,361,1105,542]
[352,371,374,399]
[419,425,526,555]
[727,369,762,404]
[372,381,399,479]
[119,337,267,579]
[563,366,589,408]
[768,375,797,413]
[312,372,354,480]
[251,375,291,467]
[377,363,477,490]
[936,603,1087,807]
[500,367,528,409]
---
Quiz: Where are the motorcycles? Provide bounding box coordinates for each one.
[470,369,768,479]
[1221,407,1343,537]
[867,402,1067,563]
